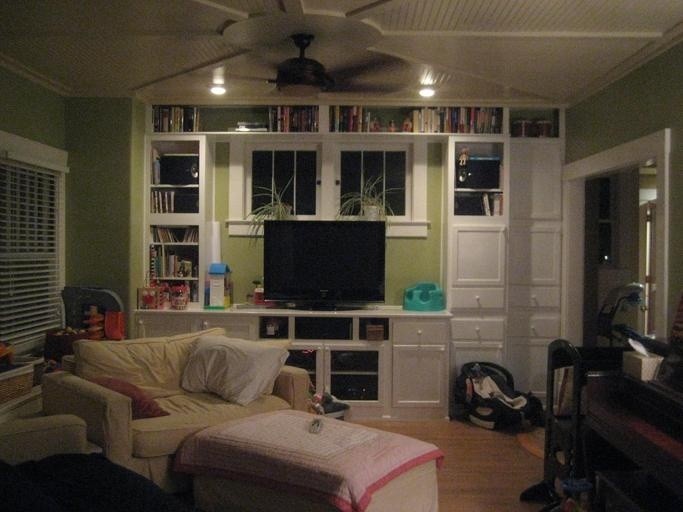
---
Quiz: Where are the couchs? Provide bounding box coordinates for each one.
[1,413,102,464]
[39,326,311,462]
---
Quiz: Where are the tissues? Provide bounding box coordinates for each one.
[623,339,664,380]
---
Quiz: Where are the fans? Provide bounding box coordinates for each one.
[184,32,413,111]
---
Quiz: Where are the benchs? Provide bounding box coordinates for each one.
[173,407,446,512]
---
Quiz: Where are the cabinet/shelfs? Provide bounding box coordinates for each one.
[511,141,563,408]
[142,99,568,143]
[446,135,509,378]
[142,134,214,311]
[131,304,454,419]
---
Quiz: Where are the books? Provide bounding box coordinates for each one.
[235,106,318,132]
[151,189,175,213]
[152,104,199,131]
[151,245,197,277]
[483,194,502,215]
[151,226,199,241]
[330,105,501,132]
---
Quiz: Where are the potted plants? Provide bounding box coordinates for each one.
[243,175,295,234]
[332,175,404,223]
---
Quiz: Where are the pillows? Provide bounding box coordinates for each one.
[86,376,173,421]
[181,333,291,408]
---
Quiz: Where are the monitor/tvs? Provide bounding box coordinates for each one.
[263,219,387,312]
